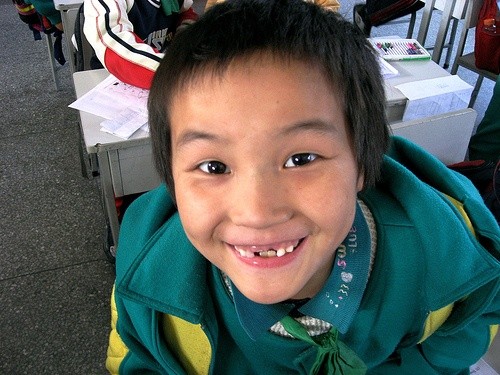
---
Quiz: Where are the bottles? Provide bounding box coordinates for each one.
[484,19,497,34]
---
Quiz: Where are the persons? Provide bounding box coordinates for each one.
[27,0,500,374]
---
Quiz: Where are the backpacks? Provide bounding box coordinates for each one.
[475,0,500,75]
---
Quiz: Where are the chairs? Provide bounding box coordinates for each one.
[62,8,104,178]
[386,109,499,375]
[451,0,500,96]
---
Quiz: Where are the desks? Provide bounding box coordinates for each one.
[54,0,472,264]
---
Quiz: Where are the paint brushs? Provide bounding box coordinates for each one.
[371,37,431,61]
